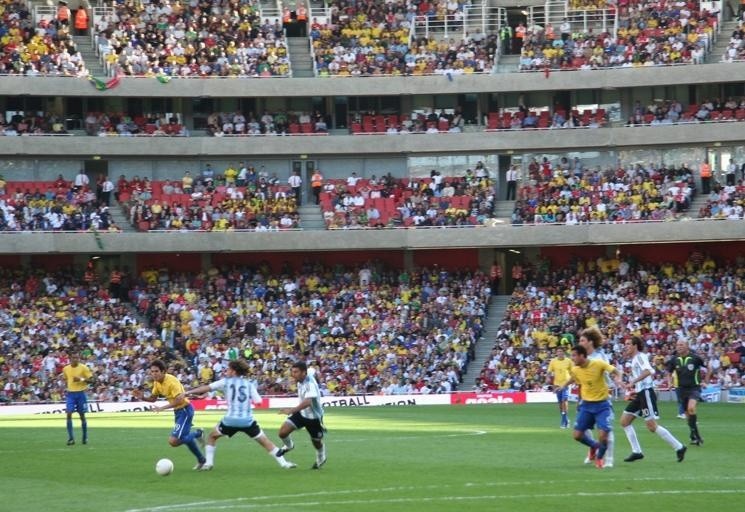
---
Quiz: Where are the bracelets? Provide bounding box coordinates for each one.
[79,377,85,382]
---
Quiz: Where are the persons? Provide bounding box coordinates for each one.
[59,351,94,445]
[276,361,328,468]
[546,346,575,428]
[578,327,614,467]
[1,157,745,231]
[553,346,623,468]
[621,335,688,462]
[174,359,298,469]
[132,359,205,470]
[656,338,713,446]
[1,95,745,137]
[2,0,745,79]
[1,264,744,404]
[670,368,687,420]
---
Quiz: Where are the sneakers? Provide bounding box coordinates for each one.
[622,452,646,463]
[312,456,327,470]
[675,444,687,461]
[192,428,213,472]
[677,412,706,447]
[585,443,614,469]
[284,462,298,469]
[560,419,570,430]
[66,437,76,446]
[276,445,294,458]
[80,436,89,445]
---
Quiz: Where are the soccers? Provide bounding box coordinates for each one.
[156,458,174,477]
